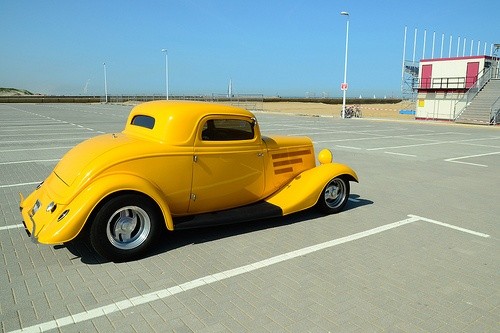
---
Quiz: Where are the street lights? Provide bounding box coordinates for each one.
[103,62,108,103]
[161,49,169,100]
[339,10,350,119]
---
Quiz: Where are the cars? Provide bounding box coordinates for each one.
[18,99,360,263]
